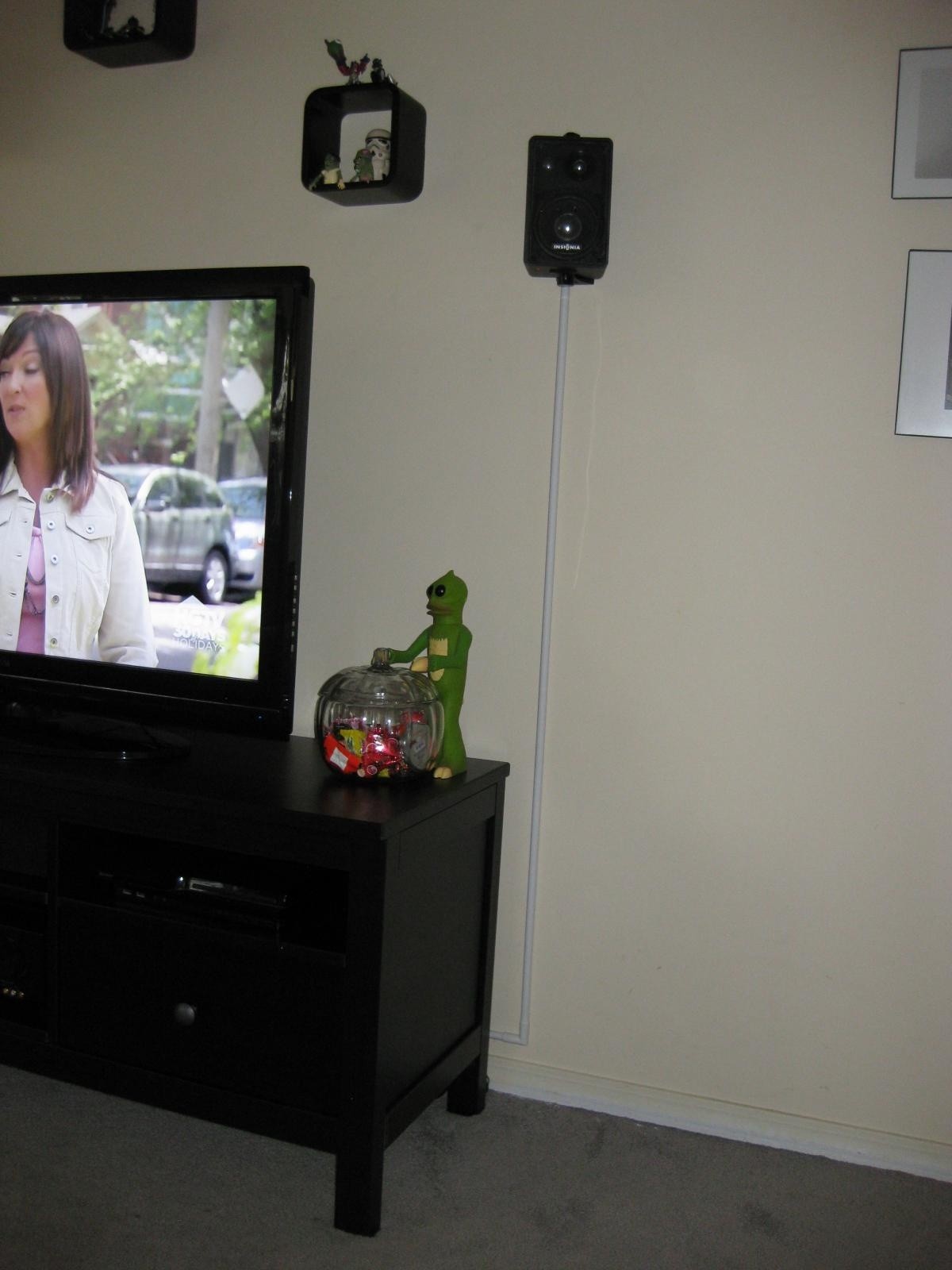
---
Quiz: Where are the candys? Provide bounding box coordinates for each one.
[324,711,428,778]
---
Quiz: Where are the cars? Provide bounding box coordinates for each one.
[216,476,268,606]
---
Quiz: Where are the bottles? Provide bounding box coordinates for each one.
[315,650,445,786]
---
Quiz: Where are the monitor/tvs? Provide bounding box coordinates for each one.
[1,260,319,760]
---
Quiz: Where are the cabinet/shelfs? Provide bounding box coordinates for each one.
[1,695,515,1238]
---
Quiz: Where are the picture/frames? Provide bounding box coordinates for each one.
[893,249,952,439]
[889,45,952,199]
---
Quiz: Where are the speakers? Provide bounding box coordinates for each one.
[523,130,614,290]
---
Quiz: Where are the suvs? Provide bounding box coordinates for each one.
[98,460,234,603]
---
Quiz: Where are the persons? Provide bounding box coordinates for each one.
[0,307,160,670]
